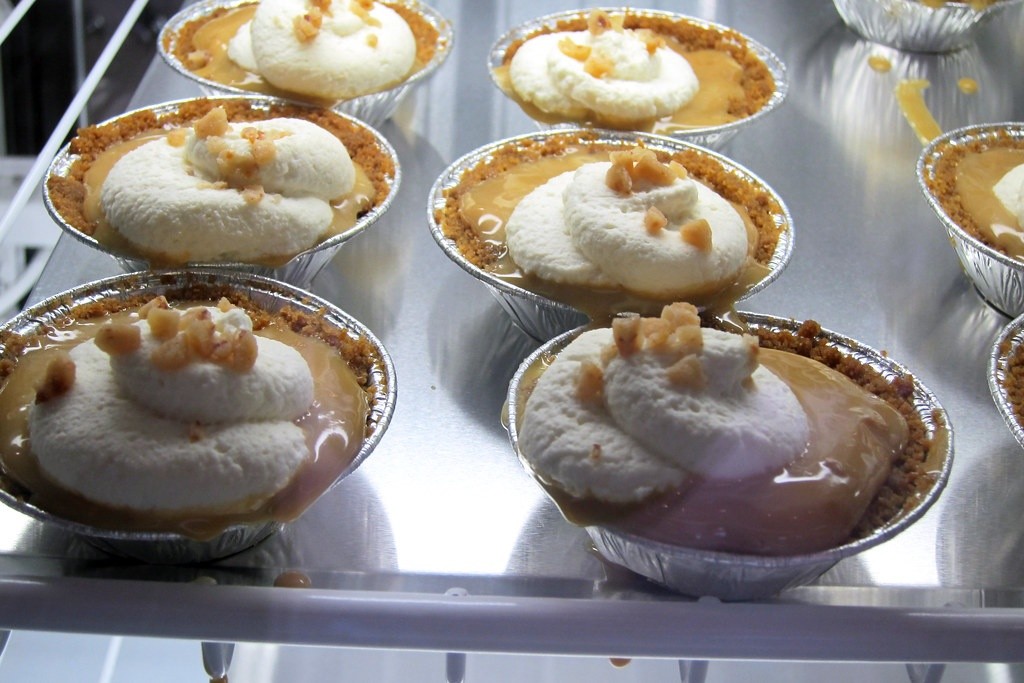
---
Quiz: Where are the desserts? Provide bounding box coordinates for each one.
[32,0,810,550]
[992,162,1024,233]
